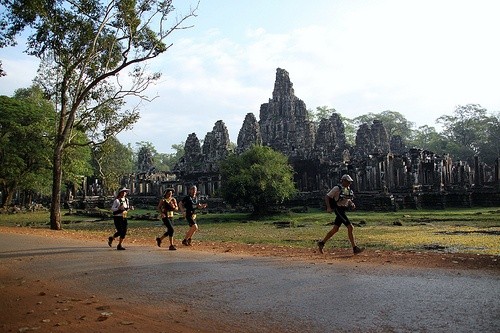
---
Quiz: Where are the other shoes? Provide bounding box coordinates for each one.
[182,241,188,246]
[188,240,193,246]
[169,245,176,250]
[108,237,115,247]
[318,241,324,254]
[353,246,365,255]
[117,246,125,250]
[156,238,161,247]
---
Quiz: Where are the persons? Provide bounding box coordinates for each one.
[108,187,134,250]
[179,185,206,246]
[156,188,178,250]
[317,174,365,255]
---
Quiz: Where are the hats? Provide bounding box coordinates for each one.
[163,188,175,197]
[119,187,131,192]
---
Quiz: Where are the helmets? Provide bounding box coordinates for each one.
[340,174,354,183]
